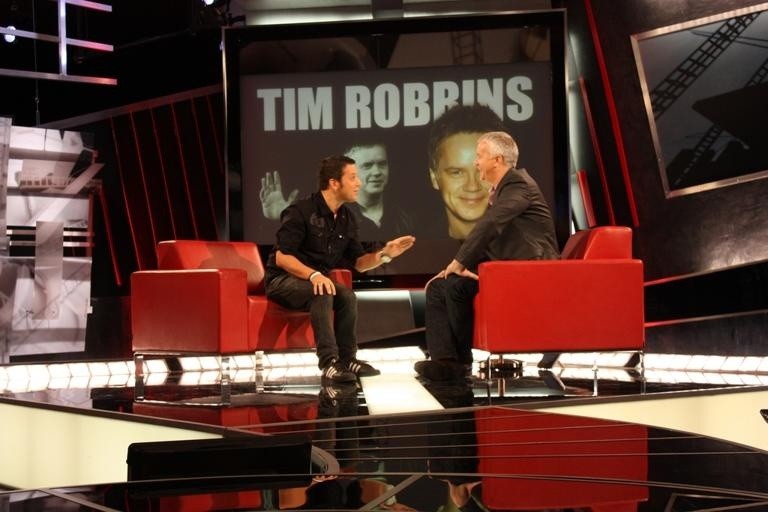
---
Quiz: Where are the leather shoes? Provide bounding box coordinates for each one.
[414,359,474,379]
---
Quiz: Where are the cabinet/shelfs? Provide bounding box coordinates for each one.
[0,117,105,365]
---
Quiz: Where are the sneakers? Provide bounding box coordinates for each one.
[321,358,356,382]
[341,355,380,377]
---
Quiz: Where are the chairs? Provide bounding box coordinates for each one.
[130,240,355,401]
[470,224,647,406]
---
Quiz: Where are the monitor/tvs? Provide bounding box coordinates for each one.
[223,8,571,291]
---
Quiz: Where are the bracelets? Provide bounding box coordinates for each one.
[377,248,392,265]
[308,270,320,282]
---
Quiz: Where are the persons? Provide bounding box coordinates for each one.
[258,389,370,510]
[258,131,414,275]
[423,102,508,273]
[413,131,560,381]
[262,152,418,383]
[414,410,493,512]
